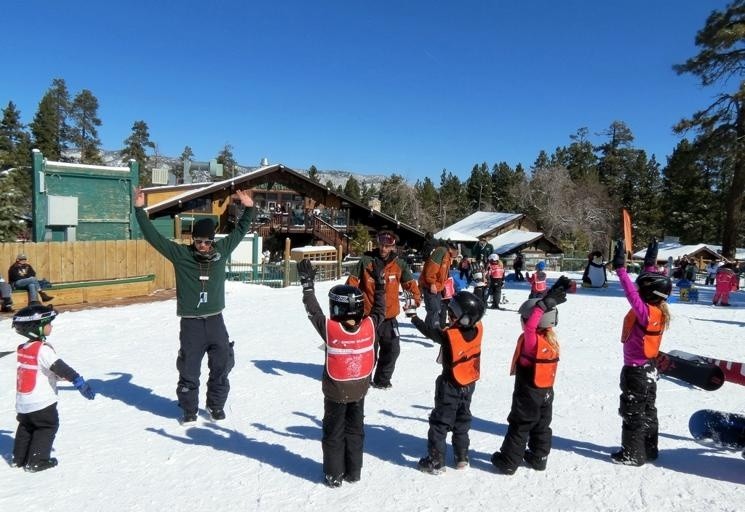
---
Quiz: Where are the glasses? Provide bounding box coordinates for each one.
[480,239,486,241]
[193,238,213,245]
[448,310,456,320]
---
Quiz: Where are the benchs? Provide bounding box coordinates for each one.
[10,274,156,309]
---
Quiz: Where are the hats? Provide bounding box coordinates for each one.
[16,254,27,261]
[193,217,216,238]
[374,230,400,246]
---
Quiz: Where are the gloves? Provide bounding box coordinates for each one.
[366,257,385,288]
[402,304,417,318]
[544,275,572,296]
[643,237,659,266]
[535,286,569,313]
[612,239,625,269]
[72,375,96,400]
[295,259,319,292]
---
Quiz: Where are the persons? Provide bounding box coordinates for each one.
[491,275,571,476]
[401,231,551,339]
[132,183,254,421]
[297,257,386,488]
[610,237,672,467]
[8,254,54,306]
[11,306,96,473]
[345,231,422,389]
[403,292,485,475]
[674,255,740,306]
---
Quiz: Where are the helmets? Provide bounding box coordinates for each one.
[517,297,559,330]
[327,284,365,323]
[487,254,499,262]
[634,271,672,306]
[447,290,486,328]
[12,305,58,340]
[535,261,545,271]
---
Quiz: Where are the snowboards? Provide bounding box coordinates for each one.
[689,410,745,450]
[655,350,745,390]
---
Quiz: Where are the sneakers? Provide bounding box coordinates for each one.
[609,447,645,467]
[321,471,342,488]
[417,454,446,475]
[491,452,518,476]
[342,473,360,484]
[183,409,197,421]
[24,458,58,473]
[453,454,470,470]
[206,404,226,420]
[12,458,25,468]
[375,381,392,389]
[523,450,547,471]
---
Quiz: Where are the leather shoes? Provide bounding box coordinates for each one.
[42,296,53,302]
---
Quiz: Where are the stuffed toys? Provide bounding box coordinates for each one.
[582,251,608,288]
